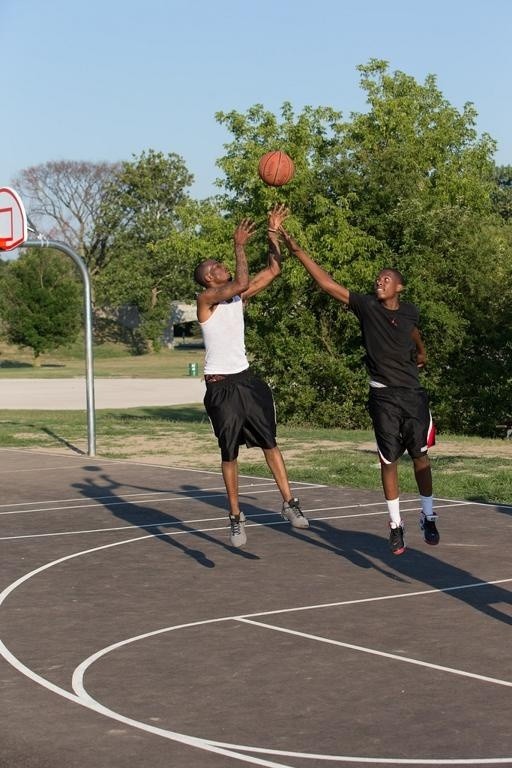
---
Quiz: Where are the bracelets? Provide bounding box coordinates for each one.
[268,228,278,233]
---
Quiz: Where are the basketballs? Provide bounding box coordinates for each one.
[258,150,294,186]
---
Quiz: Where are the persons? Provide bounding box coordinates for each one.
[193,201,310,550]
[276,223,440,557]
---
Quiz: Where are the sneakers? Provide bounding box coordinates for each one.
[388,519,408,556]
[280,497,310,529]
[417,510,440,544]
[229,511,247,548]
